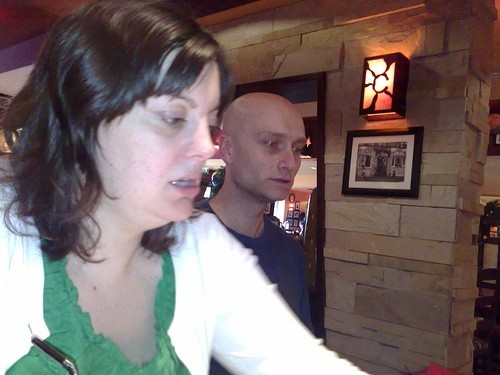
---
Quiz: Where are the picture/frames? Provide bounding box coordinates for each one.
[342,126,424,198]
[286,201,301,227]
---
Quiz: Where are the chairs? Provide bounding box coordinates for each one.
[473,267,500,375]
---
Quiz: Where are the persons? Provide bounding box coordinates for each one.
[0,0,365,375]
[195,92,314,375]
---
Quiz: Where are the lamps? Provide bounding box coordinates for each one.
[359,52,409,122]
